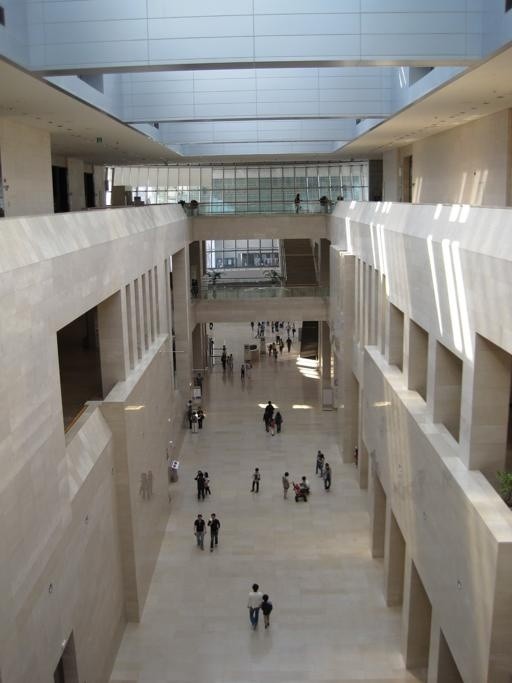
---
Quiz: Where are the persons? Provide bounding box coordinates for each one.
[207,513,221,552]
[262,594,272,628]
[295,193,301,216]
[185,319,332,502]
[193,512,207,551]
[247,583,263,631]
[140,471,153,500]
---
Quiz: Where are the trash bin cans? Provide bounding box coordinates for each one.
[172,460,179,482]
